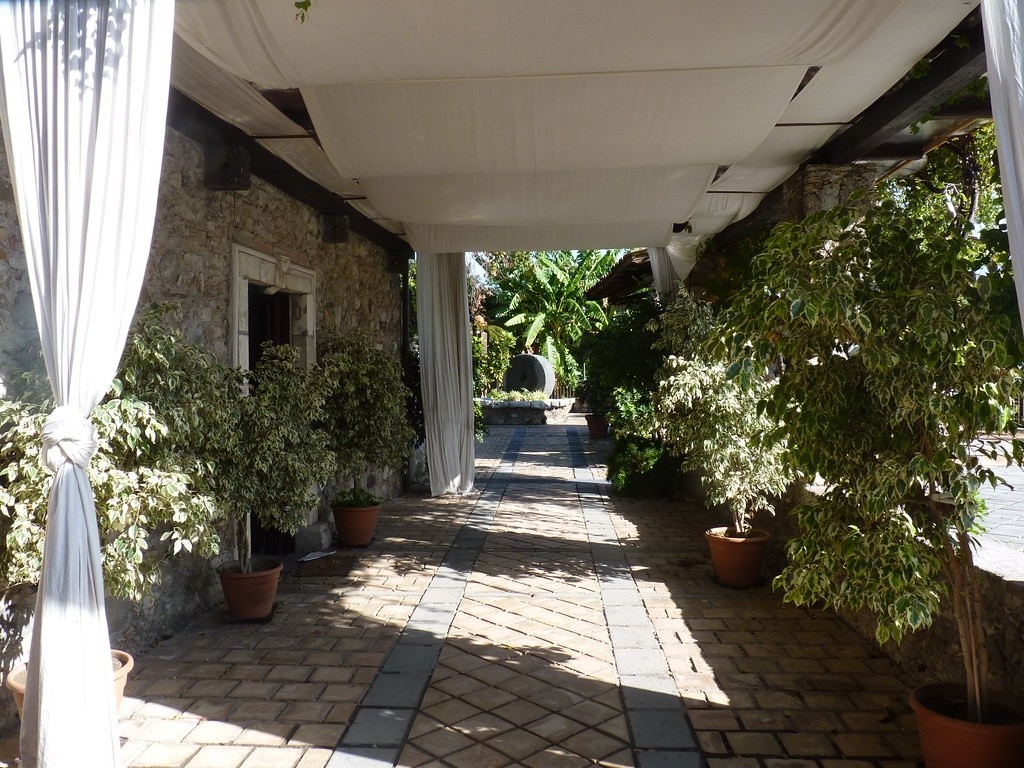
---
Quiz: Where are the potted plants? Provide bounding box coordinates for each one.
[648,276,810,591]
[603,383,681,496]
[760,124,1024,768]
[0,302,417,729]
